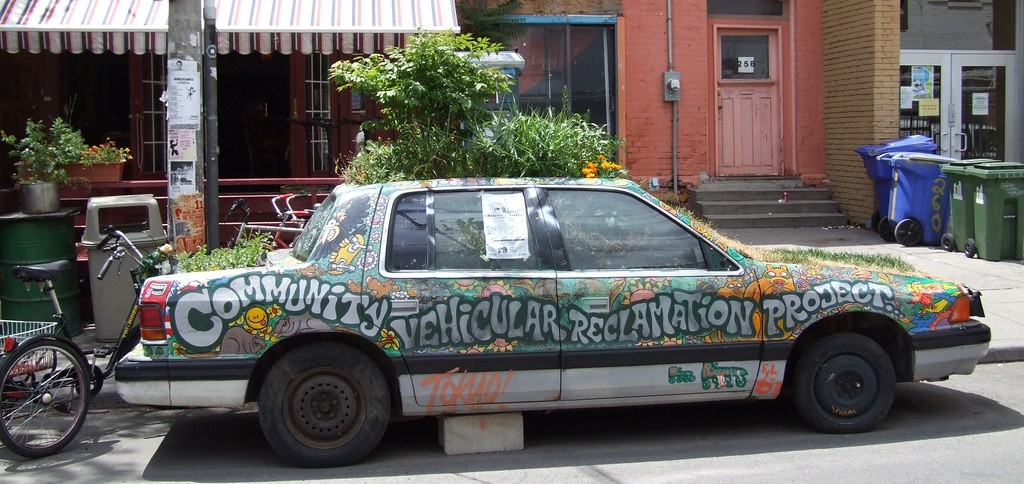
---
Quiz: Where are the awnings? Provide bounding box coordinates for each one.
[0,0,461,55]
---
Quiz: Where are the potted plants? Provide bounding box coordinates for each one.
[0,117,90,214]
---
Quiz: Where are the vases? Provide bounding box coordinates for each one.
[14,159,127,183]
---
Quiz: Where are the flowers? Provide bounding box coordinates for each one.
[81,136,133,168]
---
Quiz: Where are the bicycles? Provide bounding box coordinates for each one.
[222,189,319,254]
[0,221,178,459]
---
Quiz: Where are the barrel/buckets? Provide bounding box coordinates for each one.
[0,208,82,337]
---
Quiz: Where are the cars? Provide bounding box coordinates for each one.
[114,175,993,470]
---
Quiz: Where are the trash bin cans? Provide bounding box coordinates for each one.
[855,134,938,234]
[939,158,1002,253]
[81,194,169,344]
[960,162,1024,262]
[876,152,960,248]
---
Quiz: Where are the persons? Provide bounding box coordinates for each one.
[174,61,185,71]
